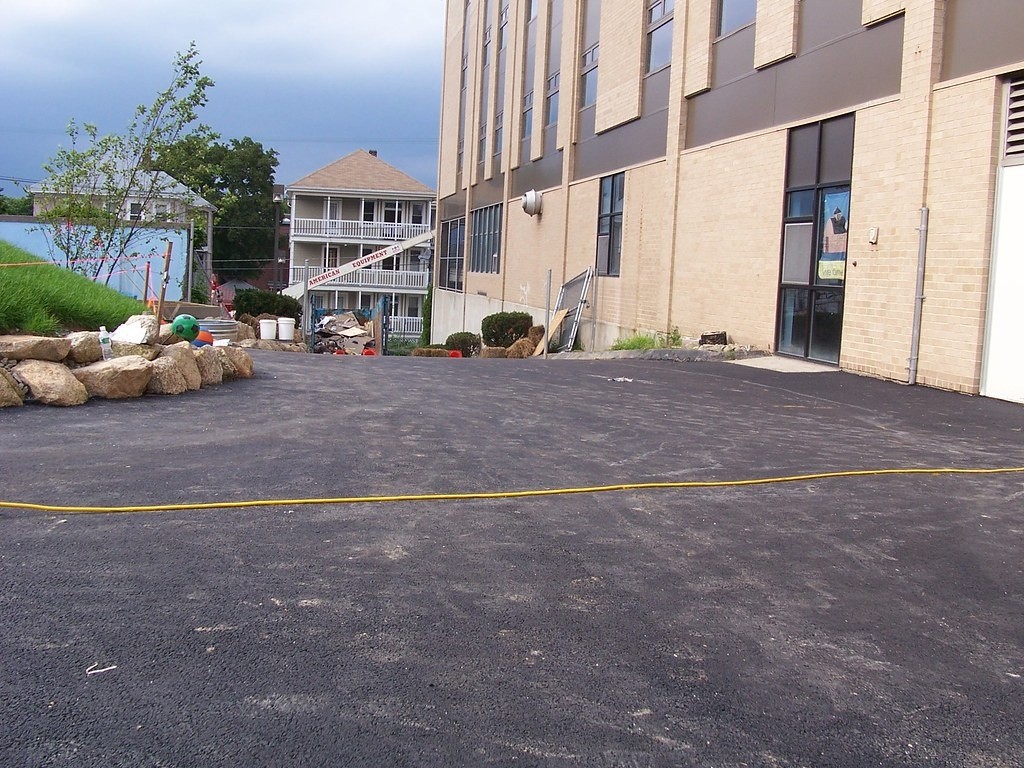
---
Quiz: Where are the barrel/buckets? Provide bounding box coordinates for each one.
[197,320,238,342]
[259,320,278,339]
[278,318,296,340]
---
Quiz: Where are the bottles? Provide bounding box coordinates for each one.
[99,326,114,361]
